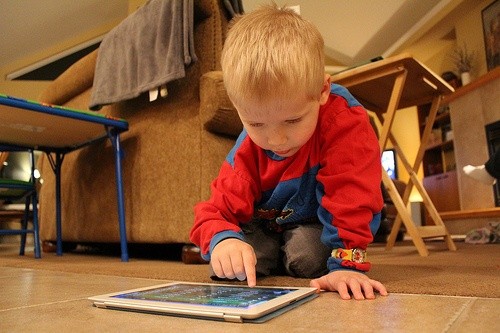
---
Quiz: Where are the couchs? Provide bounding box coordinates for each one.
[32,0,245,262]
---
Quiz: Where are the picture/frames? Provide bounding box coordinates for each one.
[481,0,500,71]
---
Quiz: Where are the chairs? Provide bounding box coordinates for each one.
[0,146,40,258]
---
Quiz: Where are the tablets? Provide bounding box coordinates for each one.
[87,281,317,320]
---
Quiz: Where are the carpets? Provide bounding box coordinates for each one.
[0,237,500,298]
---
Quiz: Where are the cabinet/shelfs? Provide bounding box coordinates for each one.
[416,72,461,227]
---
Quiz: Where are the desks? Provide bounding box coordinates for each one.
[331,53,456,257]
[0,92,129,263]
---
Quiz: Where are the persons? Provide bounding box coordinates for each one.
[189,2,389,301]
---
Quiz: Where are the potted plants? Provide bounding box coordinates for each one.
[451,40,475,86]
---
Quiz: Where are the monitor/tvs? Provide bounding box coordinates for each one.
[381,149,398,179]
